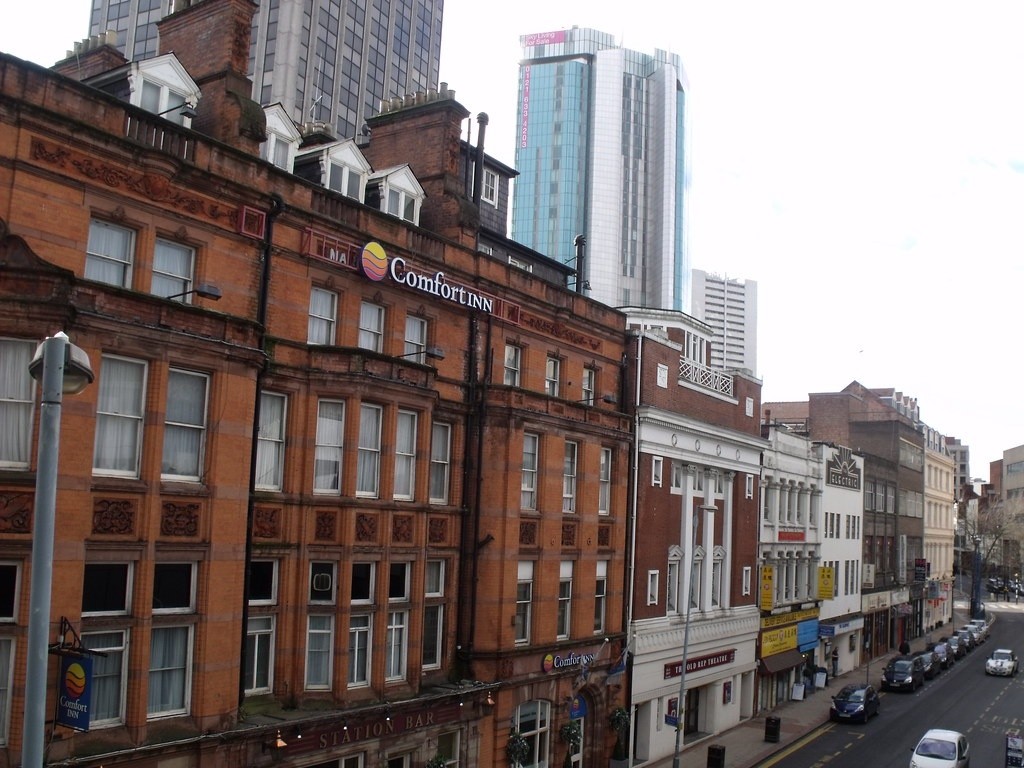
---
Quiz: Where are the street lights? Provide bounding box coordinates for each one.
[672,503,721,768]
[16,331,96,768]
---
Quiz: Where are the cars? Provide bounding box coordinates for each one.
[880,655,925,693]
[984,648,1019,676]
[939,636,967,660]
[951,630,976,650]
[970,619,991,639]
[909,729,971,768]
[924,641,955,670]
[829,682,880,725]
[911,651,941,679]
[961,625,985,645]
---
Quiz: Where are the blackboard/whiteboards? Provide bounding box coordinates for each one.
[791,682,805,700]
[814,672,827,689]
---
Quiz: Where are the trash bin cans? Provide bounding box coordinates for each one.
[706,745,726,767]
[764,716,782,743]
